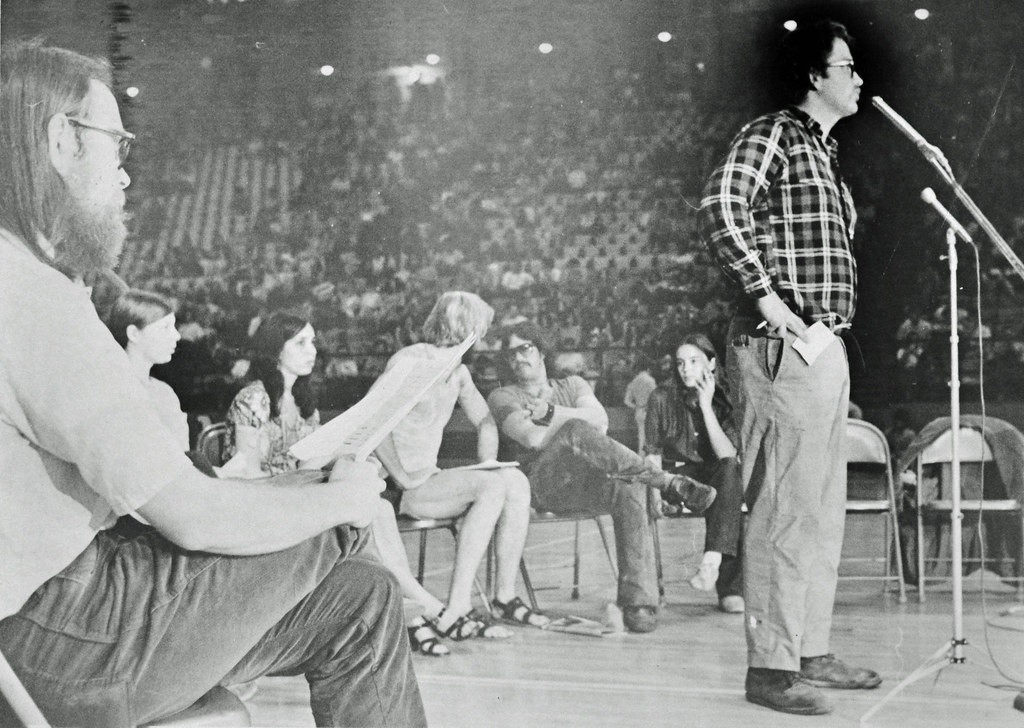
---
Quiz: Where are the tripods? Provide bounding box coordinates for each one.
[860,227,1024,725]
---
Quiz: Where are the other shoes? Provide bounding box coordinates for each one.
[797,654,882,688]
[744,667,834,715]
[719,595,744,612]
[662,474,718,512]
[690,563,719,590]
[625,606,657,634]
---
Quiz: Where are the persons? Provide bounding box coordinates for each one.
[0,40,432,728]
[370,291,548,639]
[699,23,885,717]
[643,331,745,613]
[227,314,477,658]
[487,320,716,632]
[0,39,1024,479]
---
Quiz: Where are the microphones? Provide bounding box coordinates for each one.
[919,187,972,243]
[871,95,933,159]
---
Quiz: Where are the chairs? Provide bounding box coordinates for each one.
[397,519,488,618]
[0,643,252,728]
[838,420,895,609]
[489,510,611,613]
[195,420,231,471]
[896,416,1024,609]
[647,418,748,606]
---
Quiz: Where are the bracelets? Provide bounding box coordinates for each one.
[533,402,555,427]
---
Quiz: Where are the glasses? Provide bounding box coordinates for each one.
[824,62,856,78]
[67,119,135,167]
[501,343,536,362]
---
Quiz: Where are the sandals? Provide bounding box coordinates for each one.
[423,605,474,641]
[492,597,549,628]
[466,608,513,639]
[407,623,451,657]
